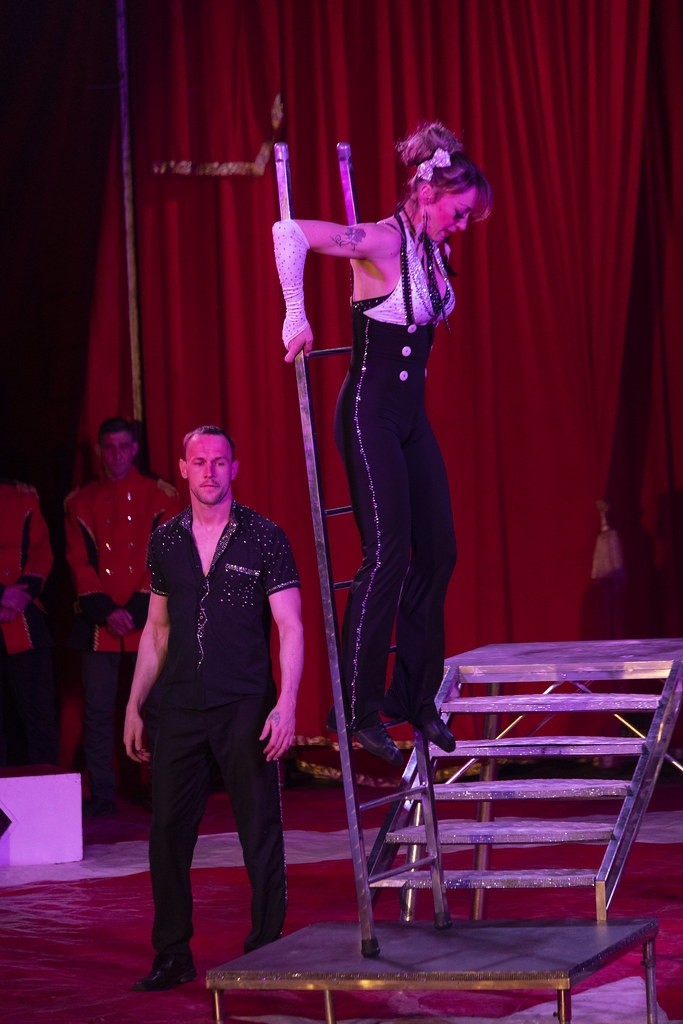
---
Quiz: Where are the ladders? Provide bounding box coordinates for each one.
[275,141,451,955]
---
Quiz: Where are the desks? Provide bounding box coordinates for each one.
[205,916,659,1024]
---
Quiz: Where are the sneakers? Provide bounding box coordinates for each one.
[324,697,461,759]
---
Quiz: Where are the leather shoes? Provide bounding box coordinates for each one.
[134,944,197,989]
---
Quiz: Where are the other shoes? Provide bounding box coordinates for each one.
[83,795,115,814]
[126,786,150,811]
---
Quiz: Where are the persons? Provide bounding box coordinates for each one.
[62,417,180,816]
[271,120,493,767]
[0,482,54,766]
[123,425,304,990]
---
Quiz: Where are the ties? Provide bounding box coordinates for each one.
[418,232,442,317]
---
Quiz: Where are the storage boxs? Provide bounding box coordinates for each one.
[0,762,83,867]
